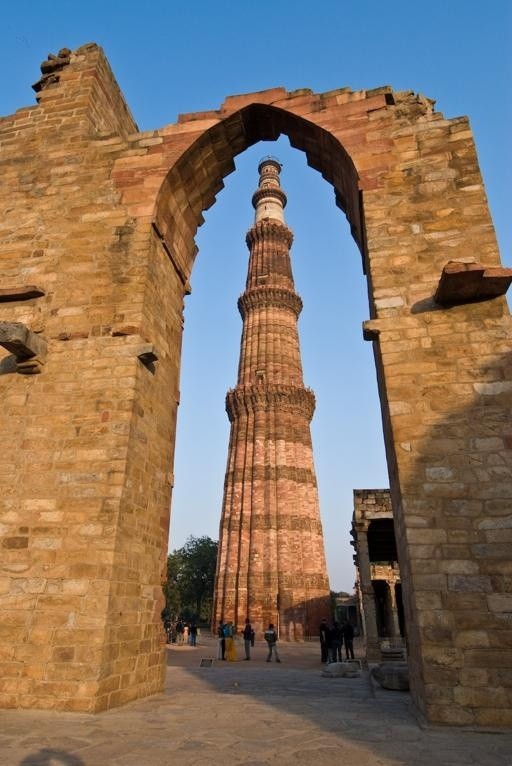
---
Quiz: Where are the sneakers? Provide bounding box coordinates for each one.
[266,659,282,663]
[243,657,251,661]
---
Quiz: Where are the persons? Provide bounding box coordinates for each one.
[341,616,355,659]
[250,627,255,647]
[329,620,345,663]
[263,622,280,662]
[223,621,241,662]
[161,616,199,647]
[319,616,330,662]
[241,617,253,660]
[215,619,224,660]
